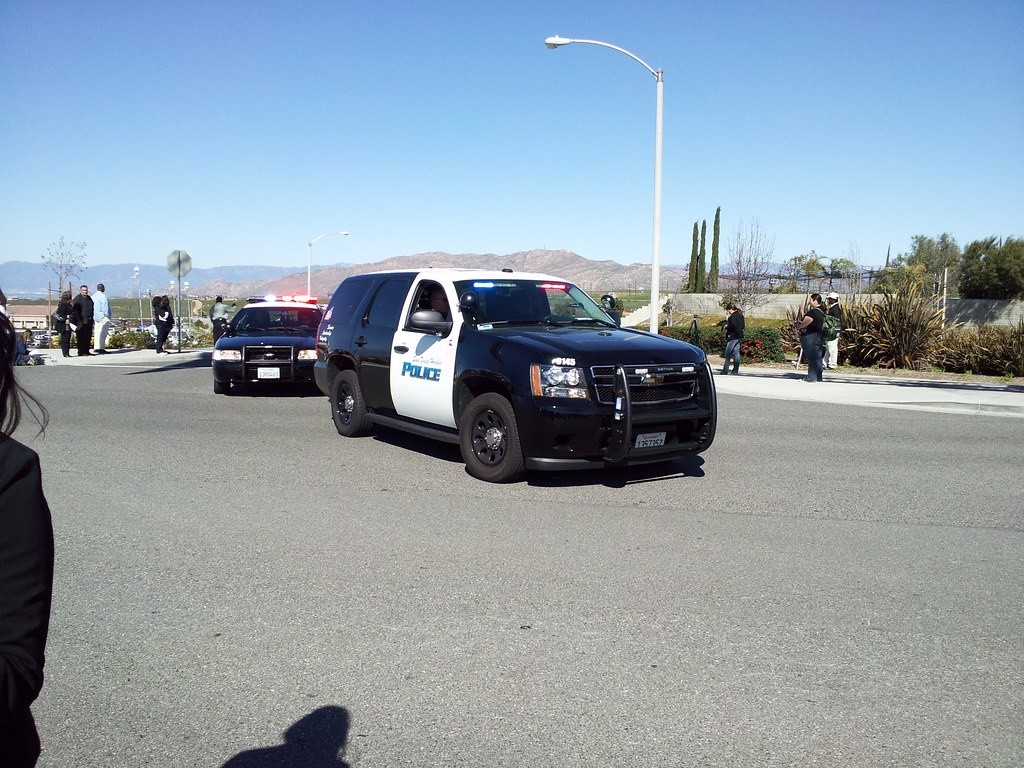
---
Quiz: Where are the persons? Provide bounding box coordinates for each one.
[606,306,620,327]
[822,292,842,370]
[422,285,449,321]
[53,291,73,358]
[720,302,745,376]
[73,285,94,356]
[210,296,237,346]
[798,294,827,384]
[90,284,111,353]
[0,288,56,768]
[297,310,318,330]
[152,296,175,355]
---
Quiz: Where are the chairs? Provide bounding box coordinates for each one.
[249,308,267,328]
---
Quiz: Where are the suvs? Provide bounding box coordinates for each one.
[312,267,718,486]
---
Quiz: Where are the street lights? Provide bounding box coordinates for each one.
[307,231,350,299]
[544,35,666,338]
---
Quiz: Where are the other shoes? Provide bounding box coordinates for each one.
[157,350,170,354]
[720,372,727,375]
[730,371,737,374]
[64,354,71,357]
[799,377,816,382]
[78,351,94,356]
[99,349,111,354]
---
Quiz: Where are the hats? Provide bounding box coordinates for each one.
[826,291,839,301]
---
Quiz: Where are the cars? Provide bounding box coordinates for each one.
[211,293,326,395]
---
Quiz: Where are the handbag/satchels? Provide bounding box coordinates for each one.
[53,304,66,322]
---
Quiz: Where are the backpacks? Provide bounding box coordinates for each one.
[814,307,839,341]
